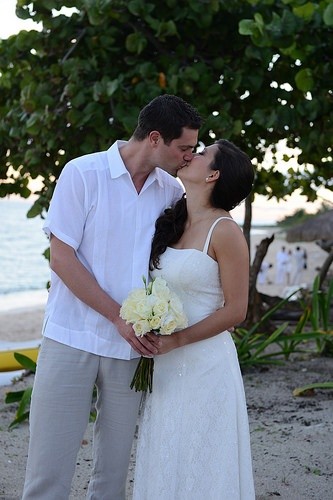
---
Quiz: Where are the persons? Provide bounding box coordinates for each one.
[21,94,205,499]
[129,138,256,499]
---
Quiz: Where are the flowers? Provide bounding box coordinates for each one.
[117,276,189,337]
